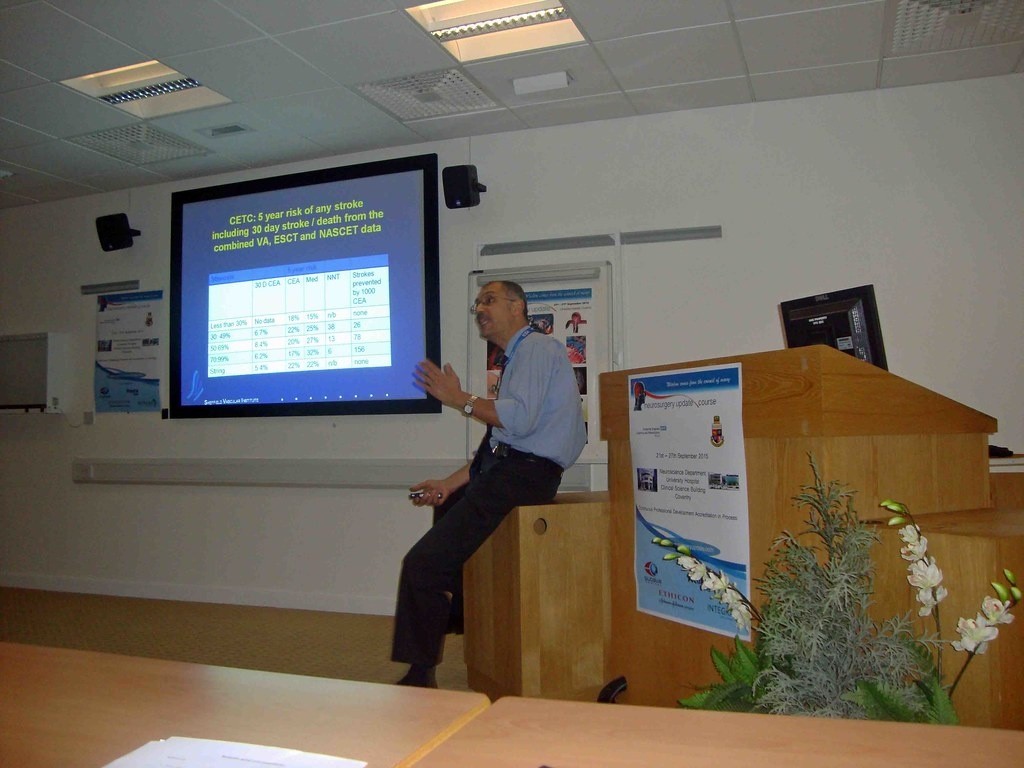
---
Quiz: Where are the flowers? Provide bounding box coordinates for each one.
[649,449,1021,724]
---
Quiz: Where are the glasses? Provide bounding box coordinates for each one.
[470,296,516,314]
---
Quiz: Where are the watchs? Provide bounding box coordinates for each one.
[462,396,479,417]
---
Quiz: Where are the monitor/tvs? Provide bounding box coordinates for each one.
[781,284,888,371]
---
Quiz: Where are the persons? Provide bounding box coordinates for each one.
[390,281,586,689]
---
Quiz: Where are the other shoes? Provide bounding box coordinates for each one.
[444,606,464,636]
[396,675,438,689]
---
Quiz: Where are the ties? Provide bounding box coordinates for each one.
[487,354,509,438]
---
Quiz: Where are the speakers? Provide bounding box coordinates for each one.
[95,213,134,252]
[442,164,480,209]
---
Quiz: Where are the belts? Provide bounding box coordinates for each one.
[495,444,563,477]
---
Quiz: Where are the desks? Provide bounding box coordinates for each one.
[0,637,1024,768]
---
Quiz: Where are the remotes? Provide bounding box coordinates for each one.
[408,492,443,499]
